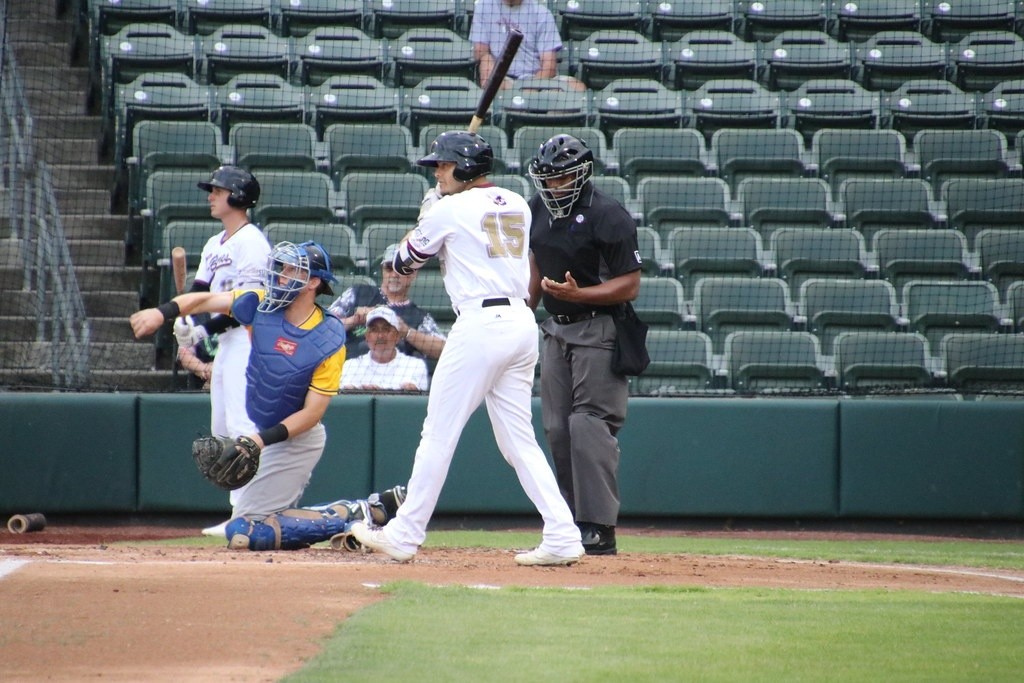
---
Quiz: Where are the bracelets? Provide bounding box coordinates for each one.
[404,327,411,340]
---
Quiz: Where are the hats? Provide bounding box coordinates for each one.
[381,244,401,265]
[366,306,400,332]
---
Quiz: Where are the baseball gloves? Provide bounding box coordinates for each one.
[192,430,261,490]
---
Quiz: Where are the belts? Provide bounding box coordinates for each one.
[552,310,608,325]
[457,298,510,316]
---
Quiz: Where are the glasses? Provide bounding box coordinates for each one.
[382,262,393,271]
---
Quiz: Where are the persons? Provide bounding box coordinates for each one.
[352,131,585,565]
[326,244,446,392]
[468,0,585,116]
[526,135,643,555]
[172,167,275,537]
[129,241,407,551]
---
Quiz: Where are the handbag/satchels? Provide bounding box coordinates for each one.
[611,303,651,378]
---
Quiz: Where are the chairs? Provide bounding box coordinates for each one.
[67,2,1023,401]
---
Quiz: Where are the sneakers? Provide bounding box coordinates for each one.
[368,486,408,525]
[350,521,415,562]
[515,544,585,567]
[582,526,617,555]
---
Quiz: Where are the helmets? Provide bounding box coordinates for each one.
[528,134,593,219]
[416,131,493,182]
[197,167,260,209]
[296,242,336,298]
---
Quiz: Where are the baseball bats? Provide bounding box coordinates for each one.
[436,29,524,199]
[172,247,187,325]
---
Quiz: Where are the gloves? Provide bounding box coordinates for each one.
[173,315,193,336]
[173,324,209,347]
[417,188,440,223]
